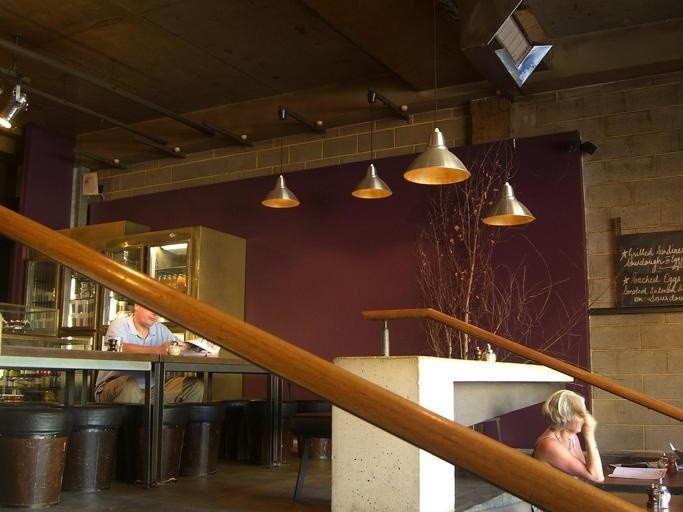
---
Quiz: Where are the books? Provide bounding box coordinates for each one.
[180,336,220,358]
[608,467,667,480]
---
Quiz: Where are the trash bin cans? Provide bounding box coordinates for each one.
[617,230,683,308]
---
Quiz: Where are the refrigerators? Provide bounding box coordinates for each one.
[97,227,244,401]
[23,218,151,350]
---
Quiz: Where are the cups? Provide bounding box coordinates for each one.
[104,336,124,352]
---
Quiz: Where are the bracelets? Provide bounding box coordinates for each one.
[585,446,598,450]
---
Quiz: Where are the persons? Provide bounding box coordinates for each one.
[531,389,605,512]
[93,303,204,403]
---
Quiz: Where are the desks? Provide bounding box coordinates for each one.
[584,469,682,511]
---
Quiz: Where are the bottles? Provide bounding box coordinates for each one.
[168,341,181,355]
[473,344,497,362]
[0,369,61,401]
[657,450,677,474]
[156,272,187,293]
[646,476,671,510]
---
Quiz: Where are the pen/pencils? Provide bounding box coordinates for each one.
[614,463,649,468]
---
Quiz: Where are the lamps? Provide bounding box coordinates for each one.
[261,107,301,209]
[481,90,537,227]
[351,91,393,199]
[402,1,471,187]
[0,79,30,130]
